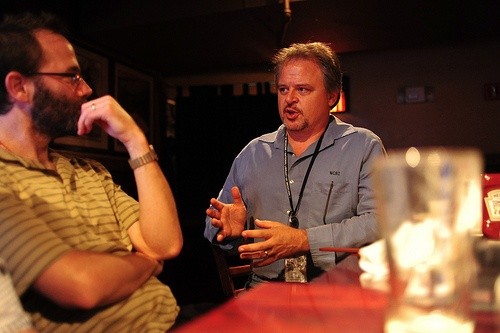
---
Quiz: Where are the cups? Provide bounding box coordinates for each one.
[370,149,483,333]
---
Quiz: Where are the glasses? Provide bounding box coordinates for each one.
[29,71,81,81]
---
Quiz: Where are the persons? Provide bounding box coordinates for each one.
[0,15,184,333]
[203,42,389,293]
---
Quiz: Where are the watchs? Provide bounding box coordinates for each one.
[127,144,162,176]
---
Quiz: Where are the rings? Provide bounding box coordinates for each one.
[90,103,97,110]
[264,251,269,258]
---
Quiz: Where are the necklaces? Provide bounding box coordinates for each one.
[0,142,11,151]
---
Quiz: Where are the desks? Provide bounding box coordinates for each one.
[168,281,500,333]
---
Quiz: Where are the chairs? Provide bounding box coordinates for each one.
[214,244,252,299]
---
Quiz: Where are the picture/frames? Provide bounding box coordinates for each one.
[111,61,155,155]
[45,44,112,154]
